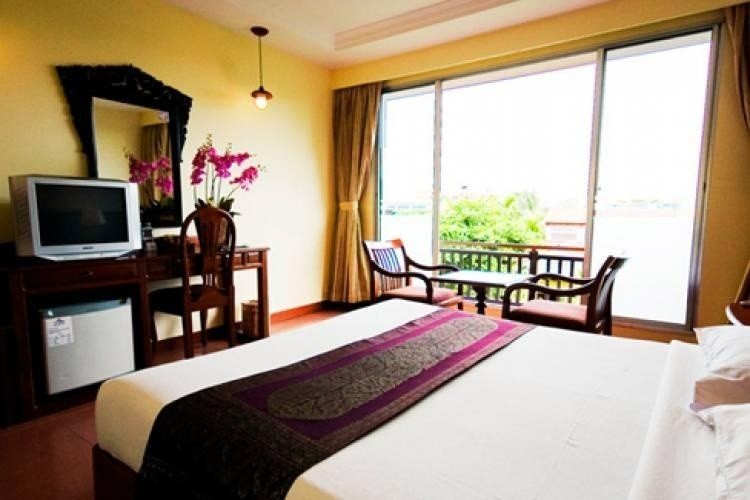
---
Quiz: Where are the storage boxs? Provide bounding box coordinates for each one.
[39,293,137,398]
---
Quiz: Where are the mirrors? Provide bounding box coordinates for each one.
[55,61,195,230]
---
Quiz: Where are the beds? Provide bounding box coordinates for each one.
[92,294,750,499]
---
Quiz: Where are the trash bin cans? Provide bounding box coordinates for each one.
[241,301,259,337]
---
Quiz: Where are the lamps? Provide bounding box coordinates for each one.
[245,24,275,112]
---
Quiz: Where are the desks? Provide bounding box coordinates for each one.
[0,238,273,418]
[426,268,537,315]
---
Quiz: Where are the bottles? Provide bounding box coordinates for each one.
[143,221,152,242]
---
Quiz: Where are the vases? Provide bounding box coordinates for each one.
[193,201,233,250]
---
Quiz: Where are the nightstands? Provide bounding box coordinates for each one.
[722,300,750,328]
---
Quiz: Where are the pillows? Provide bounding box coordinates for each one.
[693,402,750,499]
[691,322,750,407]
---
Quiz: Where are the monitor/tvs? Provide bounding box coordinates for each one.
[8,173,143,262]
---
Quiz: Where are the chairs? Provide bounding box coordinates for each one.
[360,237,464,310]
[145,204,240,364]
[501,251,628,335]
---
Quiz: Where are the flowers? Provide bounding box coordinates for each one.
[123,144,175,207]
[188,132,267,217]
